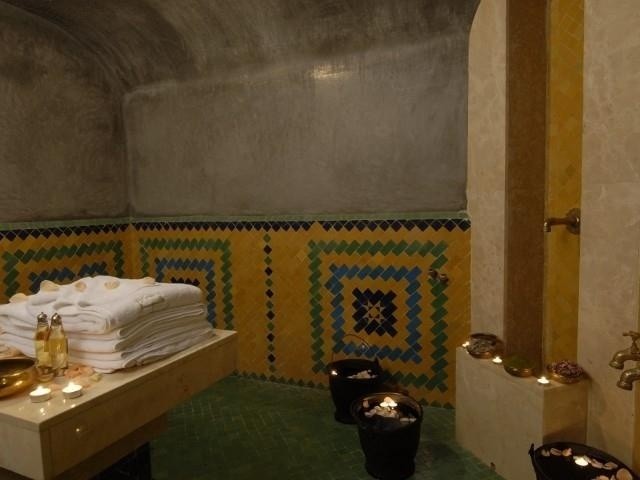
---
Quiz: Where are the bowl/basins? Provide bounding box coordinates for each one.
[1,356,39,399]
[504,358,541,378]
[468,333,498,359]
[547,361,586,384]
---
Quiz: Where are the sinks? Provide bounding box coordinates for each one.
[528,441,636,480]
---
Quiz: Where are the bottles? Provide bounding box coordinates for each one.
[34,312,55,382]
[47,312,68,369]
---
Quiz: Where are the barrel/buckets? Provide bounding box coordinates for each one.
[325,334,383,422]
[354,392,422,477]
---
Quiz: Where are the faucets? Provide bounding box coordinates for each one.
[608,330,639,368]
[616,369,639,390]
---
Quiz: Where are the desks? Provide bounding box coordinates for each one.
[0,329,239,480]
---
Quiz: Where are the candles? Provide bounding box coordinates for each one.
[62,381,83,399]
[462,340,470,348]
[537,376,550,386]
[492,356,503,364]
[29,384,51,403]
